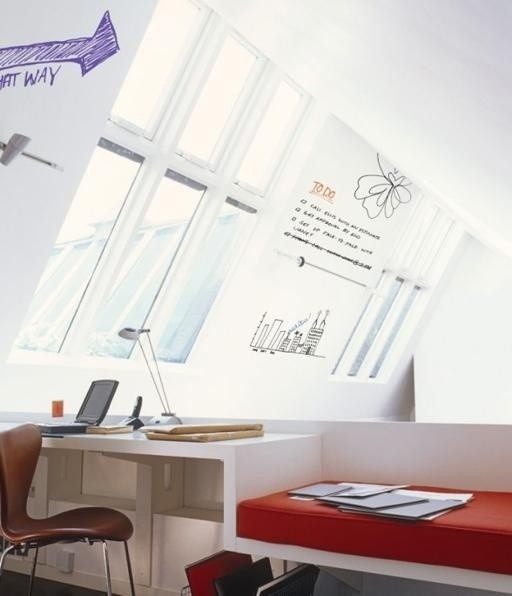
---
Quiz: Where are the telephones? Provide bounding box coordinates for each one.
[119,396,144,430]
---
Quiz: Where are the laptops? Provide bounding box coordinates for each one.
[35,379,119,434]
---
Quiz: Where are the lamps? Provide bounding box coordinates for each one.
[118,325,181,425]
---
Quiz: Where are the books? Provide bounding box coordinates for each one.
[213,557,275,596]
[183,550,254,596]
[254,562,320,596]
[285,479,475,523]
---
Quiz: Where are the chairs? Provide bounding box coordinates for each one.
[0,424,136,596]
